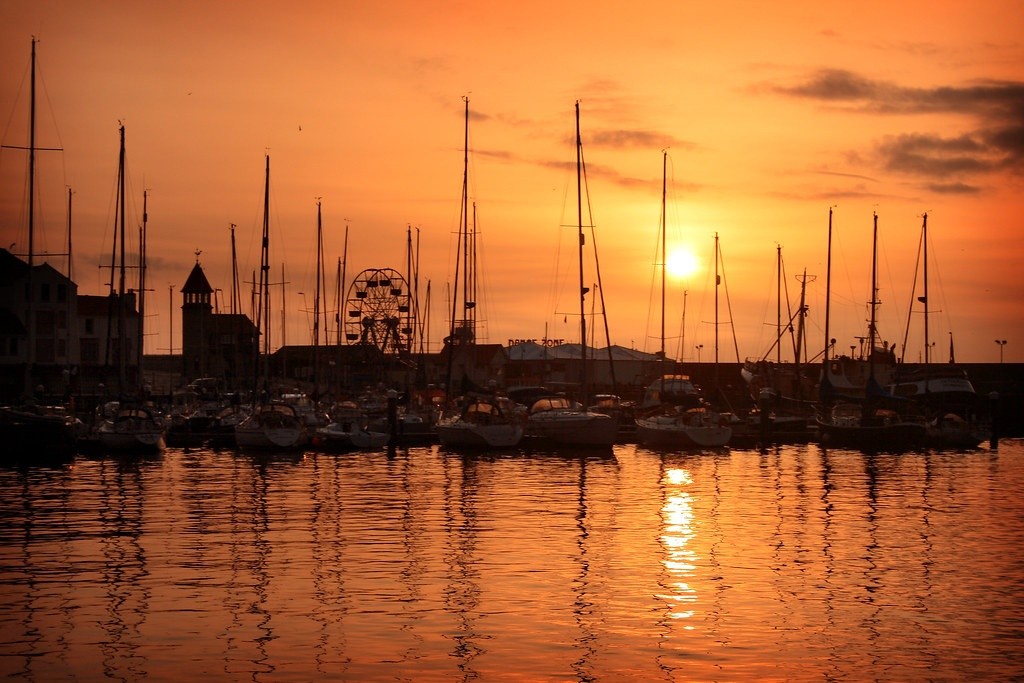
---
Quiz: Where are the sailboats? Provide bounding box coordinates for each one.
[0,34,626,454]
[634,144,990,449]
